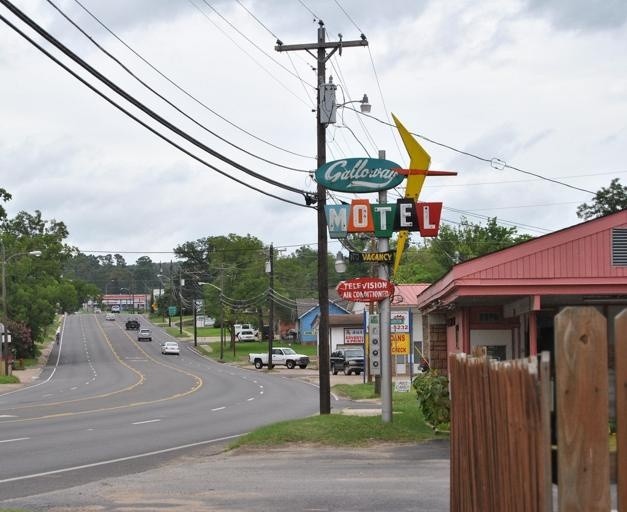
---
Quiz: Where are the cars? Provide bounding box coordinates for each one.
[111,306,120,313]
[126,320,140,330]
[234,323,262,341]
[330,348,364,375]
[160,342,179,355]
[136,329,152,341]
[106,313,115,321]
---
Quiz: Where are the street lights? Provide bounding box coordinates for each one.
[197,272,224,361]
[179,272,185,334]
[159,261,163,295]
[262,245,273,369]
[157,266,171,327]
[106,280,114,305]
[2,251,42,376]
[121,288,131,308]
[316,41,372,413]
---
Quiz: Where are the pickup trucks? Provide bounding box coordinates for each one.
[249,347,309,369]
